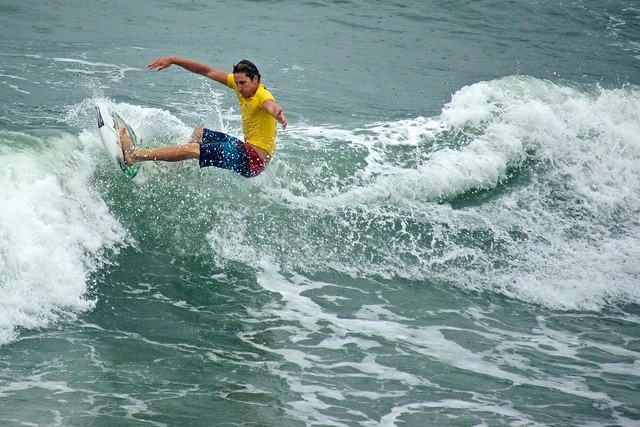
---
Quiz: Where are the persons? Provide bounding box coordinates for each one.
[120,55,288,178]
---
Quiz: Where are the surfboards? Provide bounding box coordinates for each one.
[95,102,148,180]
